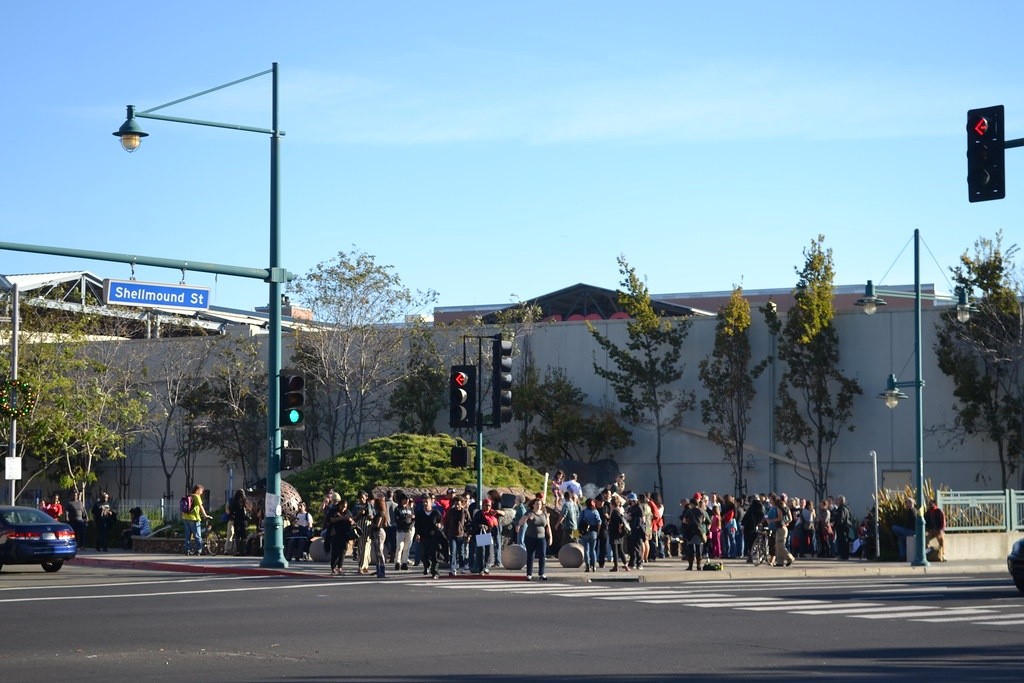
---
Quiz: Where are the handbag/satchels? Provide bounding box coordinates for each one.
[578,519,589,533]
[612,509,631,538]
[476,527,493,547]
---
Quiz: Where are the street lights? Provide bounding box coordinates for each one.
[854,229,982,567]
[112,104,289,569]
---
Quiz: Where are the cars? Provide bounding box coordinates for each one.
[1006,539,1024,594]
[0,505,78,572]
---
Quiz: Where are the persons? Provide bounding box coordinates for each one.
[892,498,917,562]
[182,485,213,555]
[858,516,870,559]
[679,492,852,570]
[121,507,150,550]
[65,490,86,550]
[284,501,313,561]
[224,488,265,556]
[41,494,63,521]
[318,469,664,581]
[924,500,946,561]
[663,523,683,558]
[92,493,113,552]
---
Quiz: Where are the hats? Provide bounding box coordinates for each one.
[332,493,341,501]
[447,488,458,494]
[694,493,702,498]
[627,493,639,501]
[482,499,491,506]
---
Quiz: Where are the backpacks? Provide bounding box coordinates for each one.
[180,494,195,513]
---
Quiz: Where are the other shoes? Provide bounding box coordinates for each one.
[773,562,791,567]
[479,571,484,576]
[526,574,531,581]
[433,573,438,580]
[424,564,431,575]
[401,563,408,570]
[583,555,703,573]
[540,573,547,580]
[338,571,345,576]
[449,570,456,576]
[359,568,370,574]
[395,564,400,570]
[331,570,337,575]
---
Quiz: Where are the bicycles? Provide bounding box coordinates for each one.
[190,518,220,556]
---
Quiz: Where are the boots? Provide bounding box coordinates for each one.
[372,566,384,577]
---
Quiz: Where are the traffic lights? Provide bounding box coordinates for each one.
[450,365,476,428]
[450,447,471,467]
[279,368,305,431]
[966,105,1005,202]
[492,339,513,428]
[282,448,304,468]
